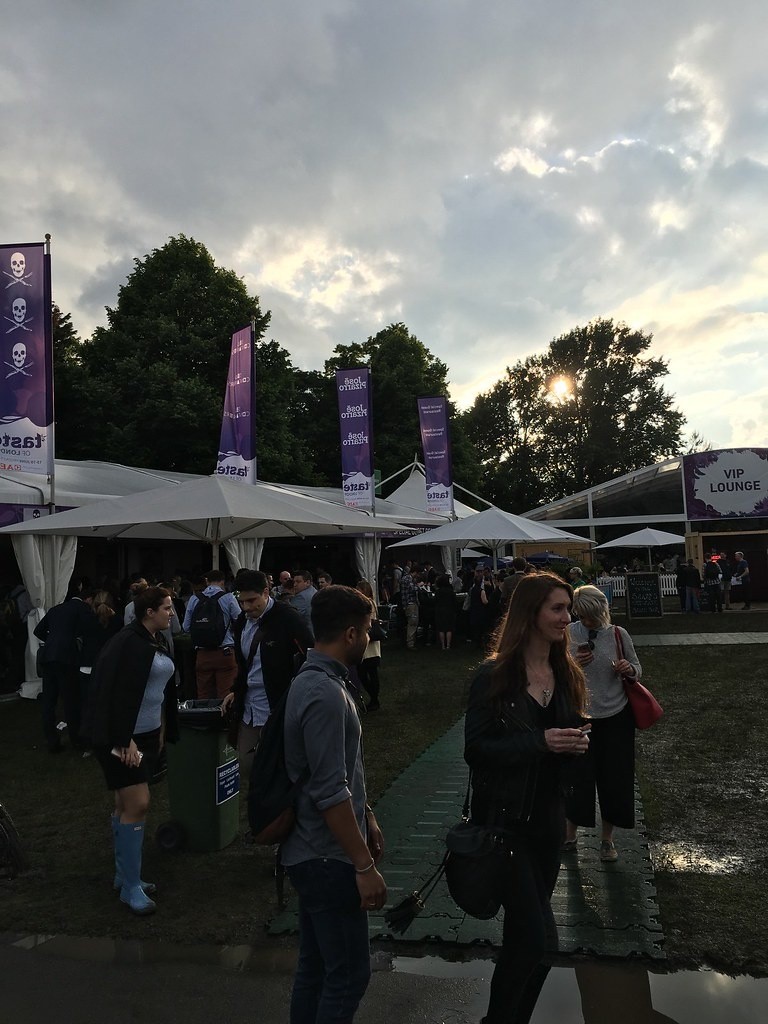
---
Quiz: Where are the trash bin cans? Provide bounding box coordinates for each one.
[166,698,240,850]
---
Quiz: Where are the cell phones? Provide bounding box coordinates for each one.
[111,745,143,767]
[578,642,591,654]
[580,730,591,734]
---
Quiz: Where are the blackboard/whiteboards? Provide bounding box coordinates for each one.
[626,572,664,619]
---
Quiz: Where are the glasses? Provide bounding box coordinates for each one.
[344,679,368,716]
[587,630,597,650]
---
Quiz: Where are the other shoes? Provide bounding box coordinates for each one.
[442,645,450,650]
[560,838,579,851]
[50,744,67,754]
[74,738,89,748]
[680,606,750,615]
[410,645,417,651]
[366,703,380,711]
[600,839,618,861]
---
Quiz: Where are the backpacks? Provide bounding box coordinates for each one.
[247,665,349,836]
[190,591,230,649]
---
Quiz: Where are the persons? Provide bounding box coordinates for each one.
[465,575,589,1024]
[469,556,541,653]
[32,569,331,913]
[565,566,585,589]
[659,551,751,613]
[356,581,380,711]
[611,564,641,572]
[563,585,643,861]
[390,557,458,650]
[283,586,388,1024]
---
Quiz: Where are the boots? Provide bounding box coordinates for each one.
[118,820,157,914]
[111,813,157,894]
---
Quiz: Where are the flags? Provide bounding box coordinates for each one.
[418,397,451,511]
[0,247,47,474]
[217,327,251,481]
[337,369,372,507]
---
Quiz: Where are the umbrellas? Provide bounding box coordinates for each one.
[461,548,489,557]
[384,507,598,570]
[0,472,419,570]
[592,527,685,571]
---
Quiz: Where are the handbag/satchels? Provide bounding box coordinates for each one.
[614,626,665,730]
[36,646,45,678]
[368,599,388,641]
[462,592,471,611]
[446,818,515,919]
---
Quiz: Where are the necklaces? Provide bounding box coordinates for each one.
[528,661,553,707]
